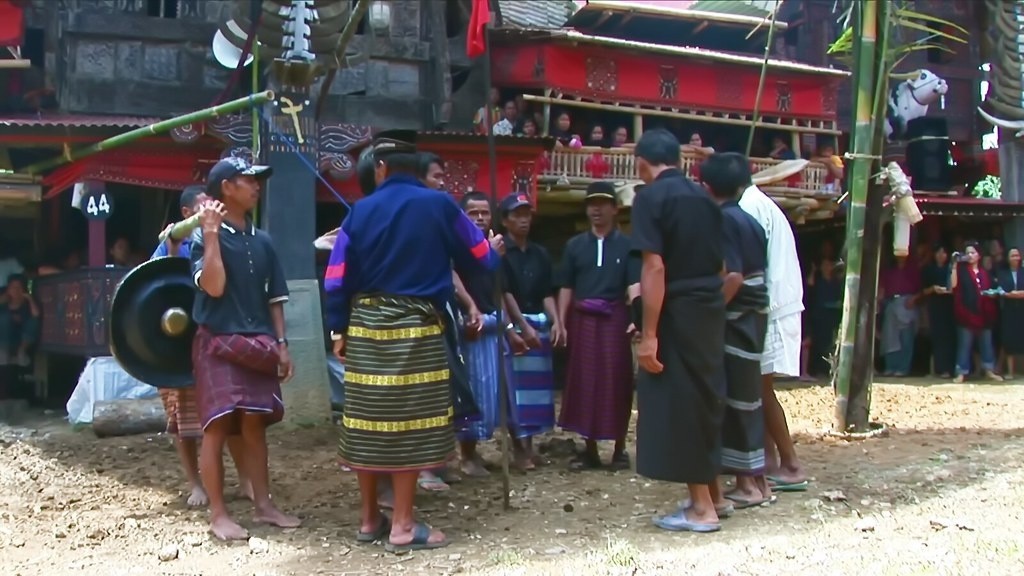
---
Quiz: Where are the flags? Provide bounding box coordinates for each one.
[466,0,489,57]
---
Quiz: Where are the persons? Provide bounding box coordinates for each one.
[472,87,844,178]
[806,223,1024,383]
[631,126,726,532]
[188,157,301,543]
[499,152,808,508]
[149,184,272,506]
[0,235,141,398]
[324,138,506,553]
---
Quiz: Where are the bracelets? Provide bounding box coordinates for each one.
[276,337,289,347]
[505,323,513,331]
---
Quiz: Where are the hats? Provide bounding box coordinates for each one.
[585,182,616,200]
[371,128,419,155]
[205,156,273,196]
[497,192,531,212]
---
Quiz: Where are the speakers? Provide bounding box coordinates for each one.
[906,117,949,191]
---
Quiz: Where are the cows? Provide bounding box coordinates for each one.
[884,69,947,144]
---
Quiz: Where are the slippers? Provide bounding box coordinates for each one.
[766,473,809,492]
[356,513,393,543]
[676,496,735,517]
[416,476,450,492]
[569,450,602,471]
[610,450,630,470]
[383,519,451,554]
[724,488,777,510]
[651,509,722,532]
